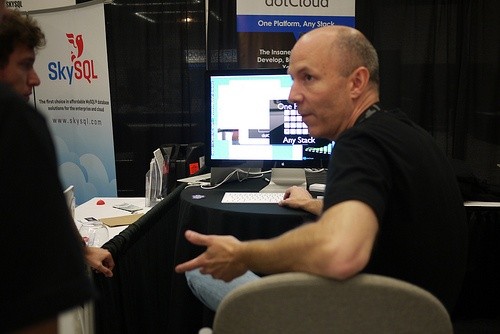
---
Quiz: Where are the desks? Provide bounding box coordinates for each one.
[172,178,319,269]
[73,196,166,250]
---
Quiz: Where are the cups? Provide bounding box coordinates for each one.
[79,221,109,248]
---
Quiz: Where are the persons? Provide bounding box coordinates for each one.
[0,9,115,334]
[175,24,471,323]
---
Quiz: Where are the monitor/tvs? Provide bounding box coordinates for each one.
[205,68,336,193]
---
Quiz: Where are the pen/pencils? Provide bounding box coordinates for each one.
[264,177,270,183]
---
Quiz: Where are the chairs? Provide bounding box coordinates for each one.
[212,272,454,334]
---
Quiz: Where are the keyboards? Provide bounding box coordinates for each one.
[220,192,285,203]
